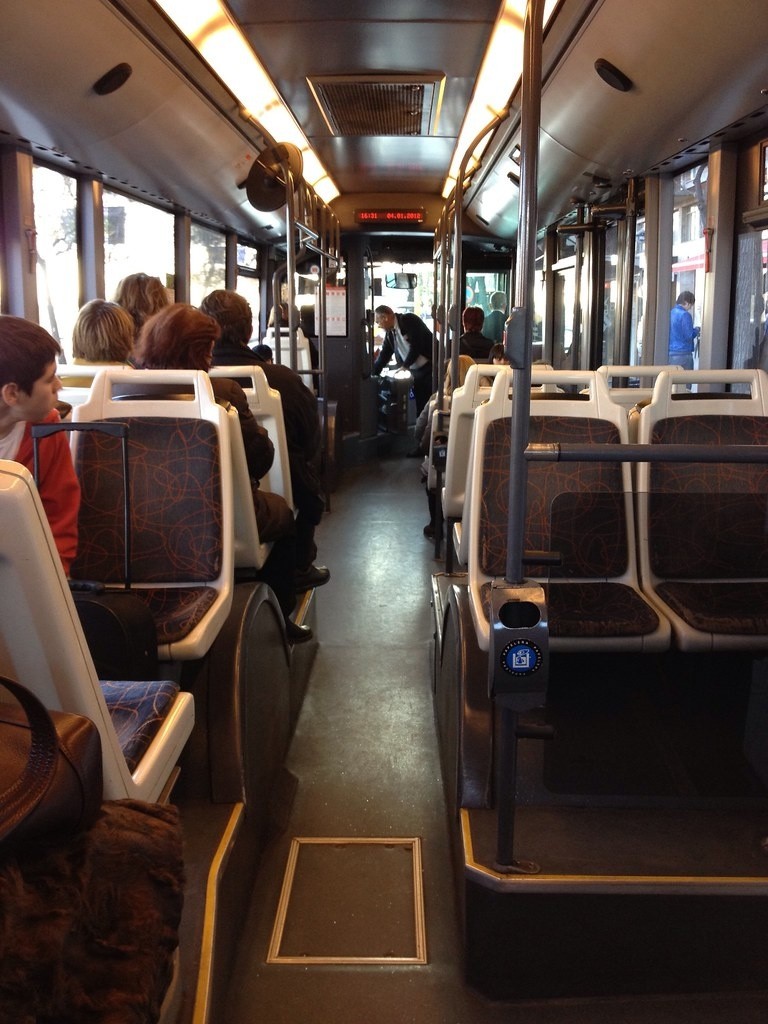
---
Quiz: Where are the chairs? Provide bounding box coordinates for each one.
[263,326,315,395]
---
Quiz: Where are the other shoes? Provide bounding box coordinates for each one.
[407,446,424,457]
[423,524,444,541]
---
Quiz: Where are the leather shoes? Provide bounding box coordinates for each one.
[290,565,331,594]
[284,615,314,646]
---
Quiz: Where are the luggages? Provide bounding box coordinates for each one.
[29,420,161,682]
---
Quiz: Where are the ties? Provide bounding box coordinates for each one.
[391,329,401,367]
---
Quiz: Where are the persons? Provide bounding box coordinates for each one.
[374,305,440,457]
[637,315,644,366]
[416,355,487,541]
[459,291,511,387]
[73,273,330,644]
[0,316,79,578]
[668,291,700,389]
[0,798,188,1024]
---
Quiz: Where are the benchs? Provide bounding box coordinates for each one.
[0,361,315,811]
[425,364,768,818]
